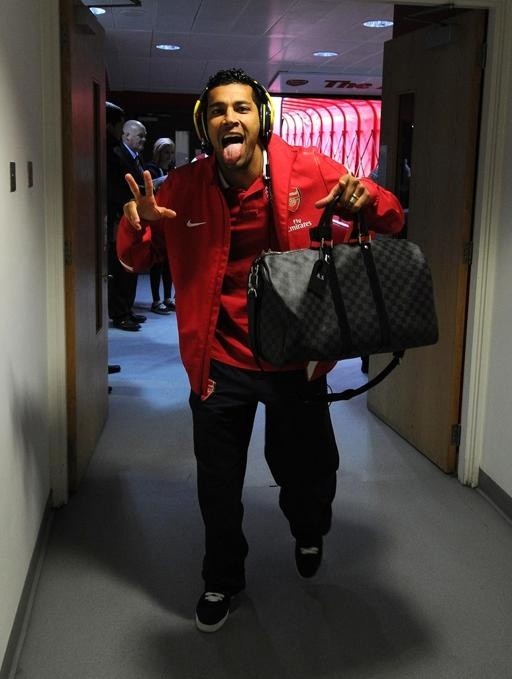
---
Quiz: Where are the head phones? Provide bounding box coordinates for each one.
[192,73,275,155]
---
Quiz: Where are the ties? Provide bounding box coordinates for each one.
[136,156,144,175]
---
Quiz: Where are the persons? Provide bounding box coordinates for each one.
[112,66,411,636]
[144,135,178,315]
[106,118,147,331]
[102,100,126,396]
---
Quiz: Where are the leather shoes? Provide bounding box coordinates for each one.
[107,365,121,374]
[129,314,147,322]
[114,320,140,330]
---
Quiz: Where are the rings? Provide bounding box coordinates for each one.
[352,193,359,200]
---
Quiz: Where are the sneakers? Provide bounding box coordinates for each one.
[151,301,178,315]
[294,534,323,581]
[195,590,231,632]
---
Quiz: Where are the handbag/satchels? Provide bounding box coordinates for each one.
[247,198,440,364]
